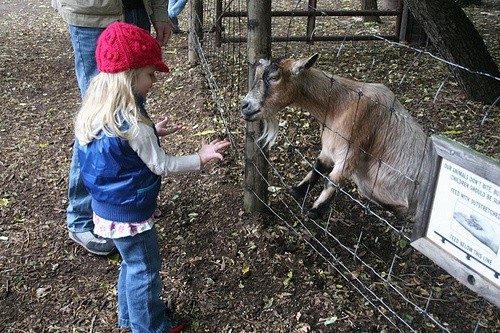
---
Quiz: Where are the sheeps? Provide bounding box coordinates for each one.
[239,52,432,221]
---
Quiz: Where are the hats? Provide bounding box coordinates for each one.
[95,21,169,74]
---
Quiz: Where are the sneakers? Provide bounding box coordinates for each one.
[167,323,185,333]
[69,229,116,255]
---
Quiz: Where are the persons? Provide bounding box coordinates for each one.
[55,0,171,257]
[73,22,234,333]
[167,0,188,33]
